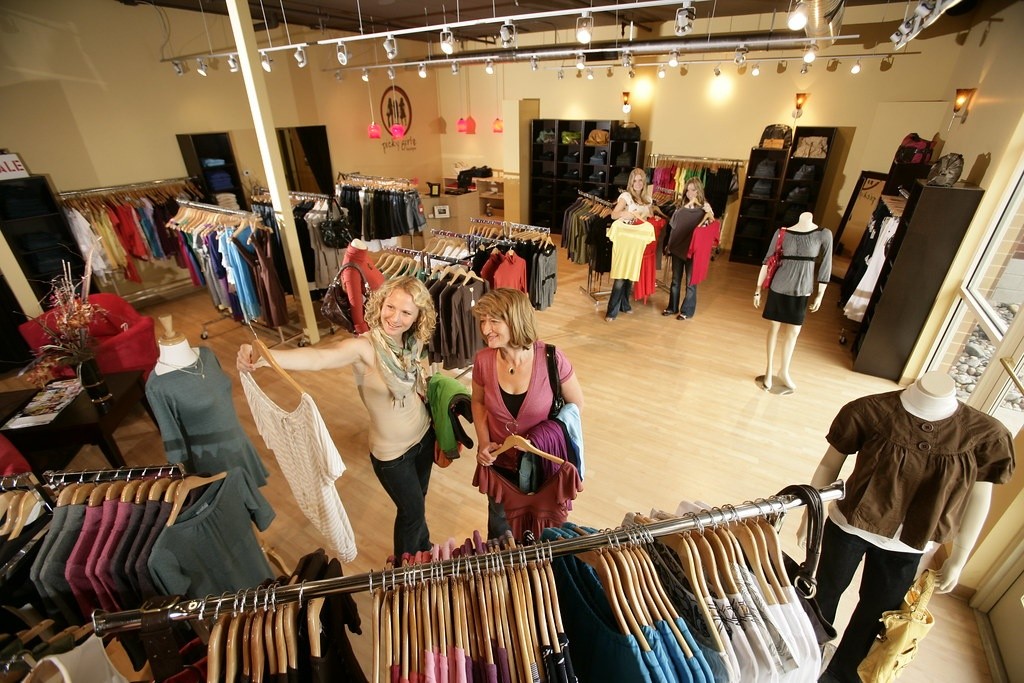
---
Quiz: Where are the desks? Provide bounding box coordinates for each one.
[0,370,162,483]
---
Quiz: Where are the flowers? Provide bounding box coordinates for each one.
[12,236,128,389]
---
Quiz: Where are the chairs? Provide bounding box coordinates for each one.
[18,293,159,381]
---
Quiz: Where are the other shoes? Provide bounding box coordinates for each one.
[623,310,633,314]
[662,309,672,316]
[605,317,612,322]
[677,313,687,321]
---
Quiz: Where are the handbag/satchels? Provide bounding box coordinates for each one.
[536,129,555,143]
[433,438,462,468]
[585,129,608,145]
[857,569,936,683]
[758,123,792,150]
[767,484,838,645]
[546,343,566,420]
[729,162,739,194]
[761,227,786,291]
[561,130,581,144]
[927,153,964,187]
[611,121,640,141]
[644,154,655,186]
[793,135,827,158]
[320,198,353,249]
[320,264,370,332]
[894,133,937,163]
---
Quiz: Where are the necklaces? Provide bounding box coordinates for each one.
[157,348,205,379]
[501,353,519,375]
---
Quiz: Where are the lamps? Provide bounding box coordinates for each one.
[151,0,994,141]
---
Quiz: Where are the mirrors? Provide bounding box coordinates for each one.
[825,171,888,284]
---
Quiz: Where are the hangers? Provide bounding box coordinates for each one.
[246,318,305,395]
[645,154,747,173]
[576,189,616,221]
[651,185,715,228]
[59,175,418,245]
[1,462,792,683]
[489,422,565,467]
[374,218,555,287]
[619,209,644,223]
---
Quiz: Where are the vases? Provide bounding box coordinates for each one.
[70,358,112,404]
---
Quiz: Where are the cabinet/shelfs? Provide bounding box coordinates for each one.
[729,124,838,267]
[274,125,334,196]
[850,178,986,384]
[527,120,646,235]
[442,174,521,225]
[838,147,935,309]
[176,131,250,211]
[0,174,103,377]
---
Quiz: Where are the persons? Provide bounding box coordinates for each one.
[145,334,277,533]
[797,371,1015,683]
[237,275,456,569]
[470,288,584,541]
[754,212,833,389]
[340,238,383,338]
[604,168,659,323]
[662,177,714,321]
